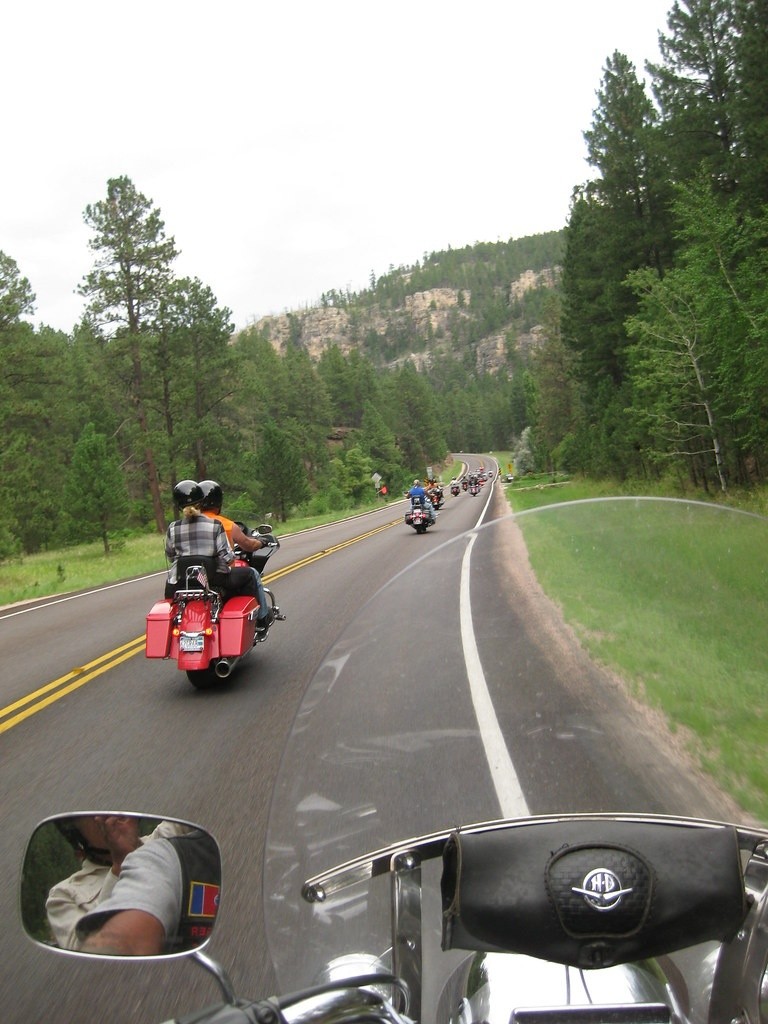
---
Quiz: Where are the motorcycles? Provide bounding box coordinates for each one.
[424,487,445,510]
[470,485,480,495]
[404,490,438,534]
[468,469,493,484]
[451,483,461,496]
[145,524,286,689]
[461,481,468,491]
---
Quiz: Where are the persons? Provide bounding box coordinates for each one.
[44,814,221,956]
[404,466,494,524]
[164,479,279,633]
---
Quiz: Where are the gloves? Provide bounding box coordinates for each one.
[257,537,270,549]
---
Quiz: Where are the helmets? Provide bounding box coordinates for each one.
[55,818,86,851]
[197,480,223,508]
[173,479,204,508]
[429,474,478,481]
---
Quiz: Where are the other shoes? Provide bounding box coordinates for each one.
[258,607,273,627]
